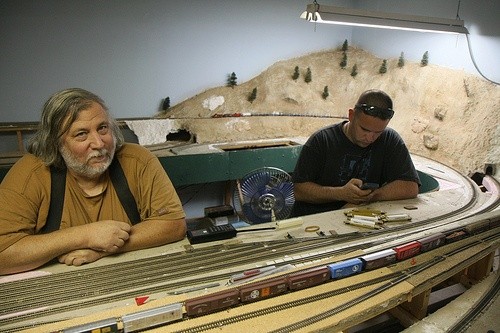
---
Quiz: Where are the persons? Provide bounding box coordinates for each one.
[0,87,191,281]
[287,88,421,219]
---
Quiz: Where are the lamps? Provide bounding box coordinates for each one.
[299,0,470,35]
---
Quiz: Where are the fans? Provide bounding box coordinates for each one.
[232,166,295,226]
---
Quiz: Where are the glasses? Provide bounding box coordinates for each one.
[355,103,395,120]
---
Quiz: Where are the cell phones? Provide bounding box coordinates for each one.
[361,182,379,191]
